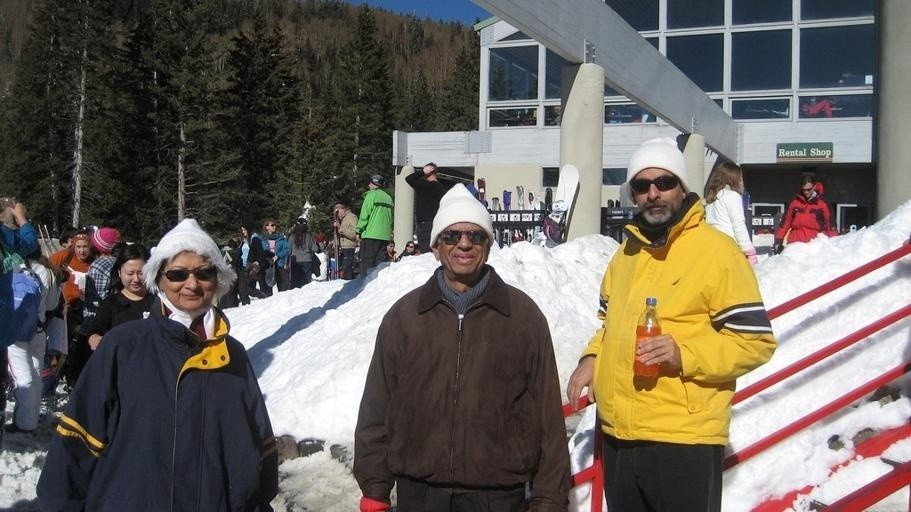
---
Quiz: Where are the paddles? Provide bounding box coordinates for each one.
[38,224,59,256]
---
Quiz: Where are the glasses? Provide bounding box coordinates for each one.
[629,175,679,194]
[159,267,217,282]
[439,229,489,245]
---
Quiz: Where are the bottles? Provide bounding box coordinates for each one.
[633,297,666,379]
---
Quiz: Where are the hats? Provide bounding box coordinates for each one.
[59,225,119,253]
[241,222,253,232]
[143,217,238,300]
[429,182,494,249]
[626,136,690,205]
[371,174,383,186]
[333,203,345,214]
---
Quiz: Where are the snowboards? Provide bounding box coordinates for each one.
[550,165,580,246]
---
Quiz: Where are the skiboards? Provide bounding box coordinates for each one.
[475,178,552,249]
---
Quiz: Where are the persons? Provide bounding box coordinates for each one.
[399,241,415,257]
[349,182,573,511]
[702,155,761,264]
[769,168,841,257]
[218,203,359,308]
[354,174,395,281]
[41,219,281,512]
[386,240,395,260]
[2,197,155,433]
[404,160,447,253]
[565,130,780,512]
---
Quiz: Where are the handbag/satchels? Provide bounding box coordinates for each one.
[0,265,42,348]
[265,268,277,287]
